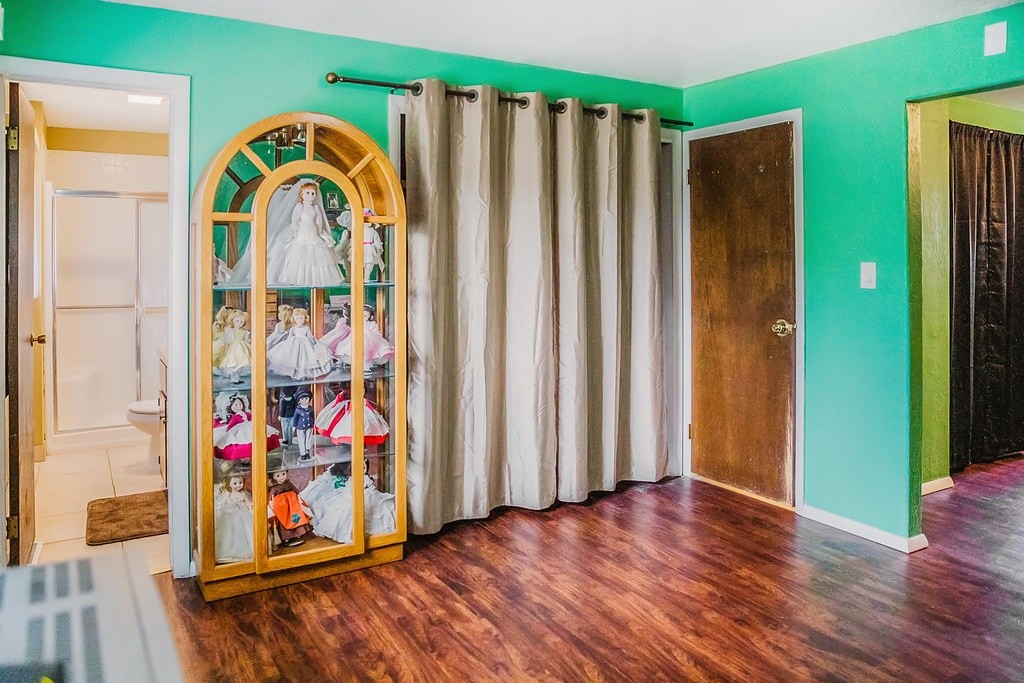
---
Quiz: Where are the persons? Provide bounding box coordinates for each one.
[266,308,333,378]
[221,311,252,385]
[213,306,233,376]
[213,390,282,466]
[315,383,352,434]
[292,388,316,461]
[277,386,297,447]
[214,465,280,565]
[267,457,314,546]
[333,209,351,284]
[312,457,394,544]
[330,380,390,452]
[347,208,385,283]
[335,305,394,373]
[230,178,346,288]
[318,303,350,354]
[265,303,293,351]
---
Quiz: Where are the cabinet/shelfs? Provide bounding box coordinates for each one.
[178,113,412,602]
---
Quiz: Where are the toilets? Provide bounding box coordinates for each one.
[125,349,162,474]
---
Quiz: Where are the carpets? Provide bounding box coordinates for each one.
[86,489,170,545]
